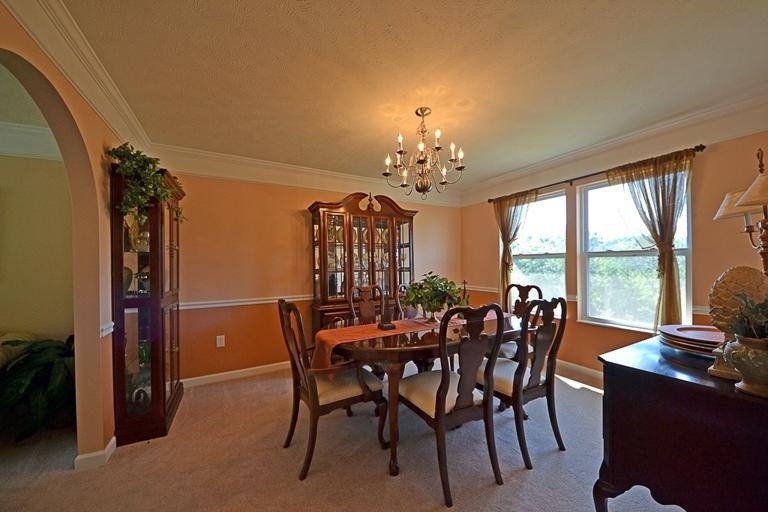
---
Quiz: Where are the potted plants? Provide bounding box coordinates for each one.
[400,269,469,326]
[722,293,767,398]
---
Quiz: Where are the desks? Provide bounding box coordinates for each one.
[594,335,767,512]
[315,306,536,477]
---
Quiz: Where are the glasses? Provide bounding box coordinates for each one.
[381,106,466,199]
[712,148,767,275]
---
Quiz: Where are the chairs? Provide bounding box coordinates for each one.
[458,297,567,470]
[350,283,393,382]
[389,303,504,507]
[486,284,544,411]
[277,283,391,480]
[396,285,455,375]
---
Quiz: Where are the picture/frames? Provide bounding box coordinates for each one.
[594,335,767,512]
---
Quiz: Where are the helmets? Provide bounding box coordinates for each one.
[658,323,737,359]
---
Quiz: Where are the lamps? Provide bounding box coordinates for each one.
[381,106,466,199]
[712,148,767,275]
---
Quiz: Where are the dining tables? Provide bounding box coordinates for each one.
[722,293,767,398]
[400,269,469,326]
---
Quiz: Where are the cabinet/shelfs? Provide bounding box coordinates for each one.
[305,191,419,348]
[109,161,186,447]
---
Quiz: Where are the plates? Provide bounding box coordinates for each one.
[305,191,419,348]
[109,161,186,447]
